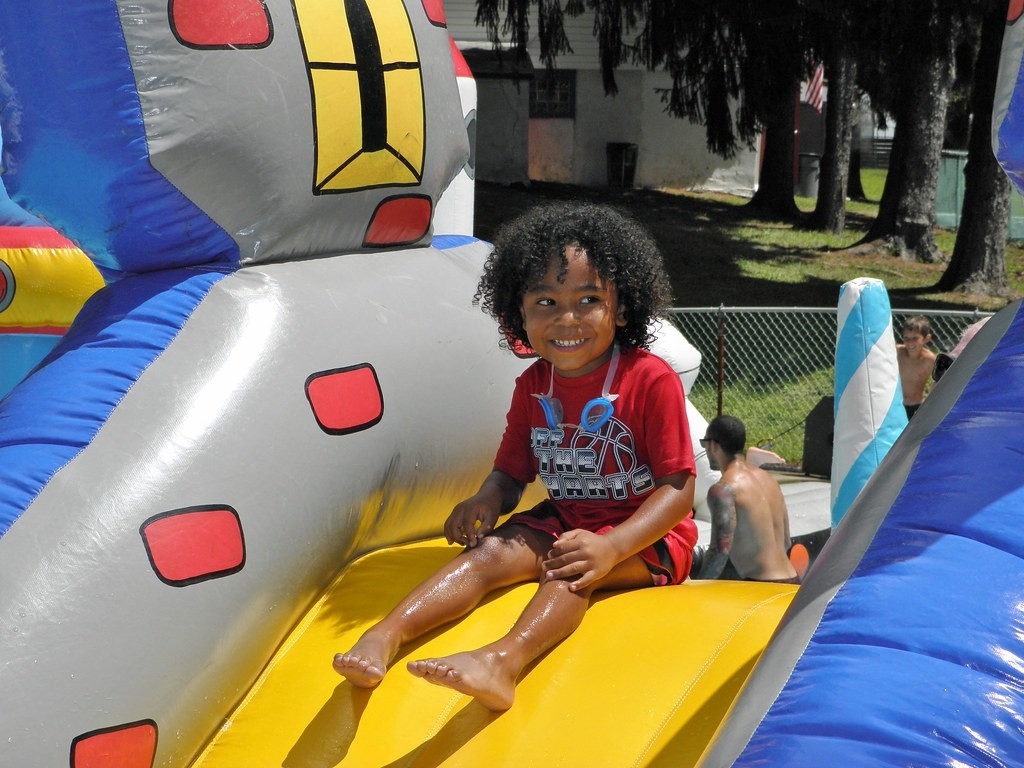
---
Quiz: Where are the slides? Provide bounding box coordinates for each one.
[172,522,803,768]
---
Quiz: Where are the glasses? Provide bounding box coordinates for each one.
[699,438,720,448]
[530,345,623,433]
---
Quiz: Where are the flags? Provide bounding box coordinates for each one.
[803,57,823,114]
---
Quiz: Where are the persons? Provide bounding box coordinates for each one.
[332,200,698,713]
[699,417,804,582]
[897,316,938,418]
